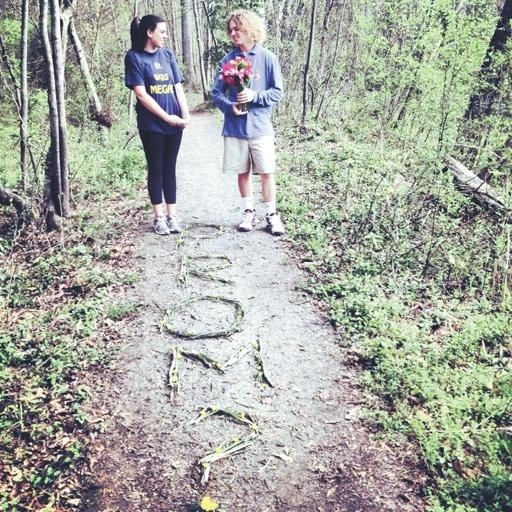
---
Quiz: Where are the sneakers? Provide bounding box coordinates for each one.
[266,213,285,236]
[153,218,181,235]
[239,209,256,231]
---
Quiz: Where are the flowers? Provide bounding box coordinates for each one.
[219,56,260,113]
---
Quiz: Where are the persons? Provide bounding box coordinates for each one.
[209,8,287,238]
[123,13,195,237]
